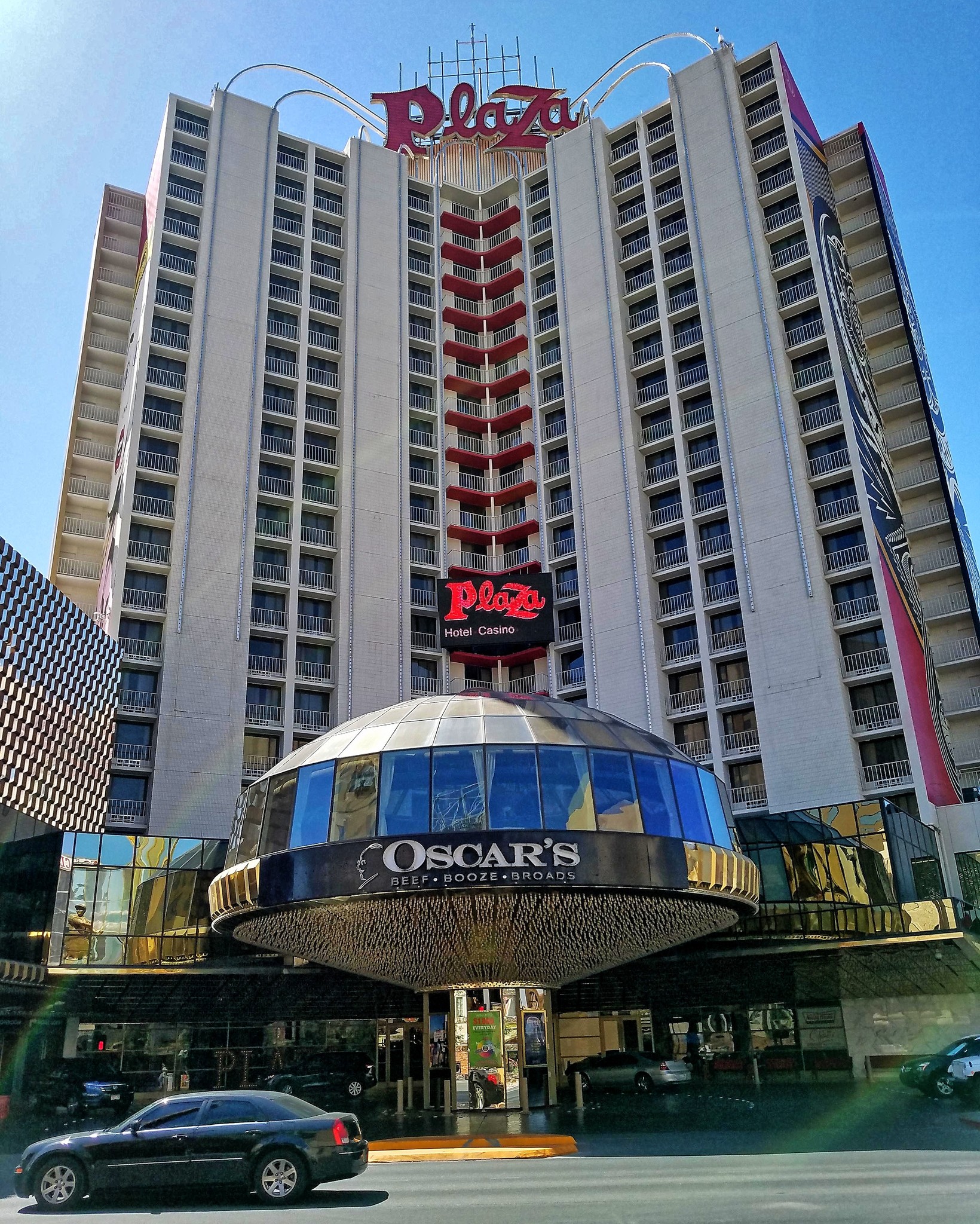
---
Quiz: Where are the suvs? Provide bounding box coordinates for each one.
[19,1056,134,1119]
[268,1049,378,1098]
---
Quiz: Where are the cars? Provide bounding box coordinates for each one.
[944,1051,980,1105]
[899,1033,980,1101]
[10,1090,370,1211]
[568,1050,692,1094]
